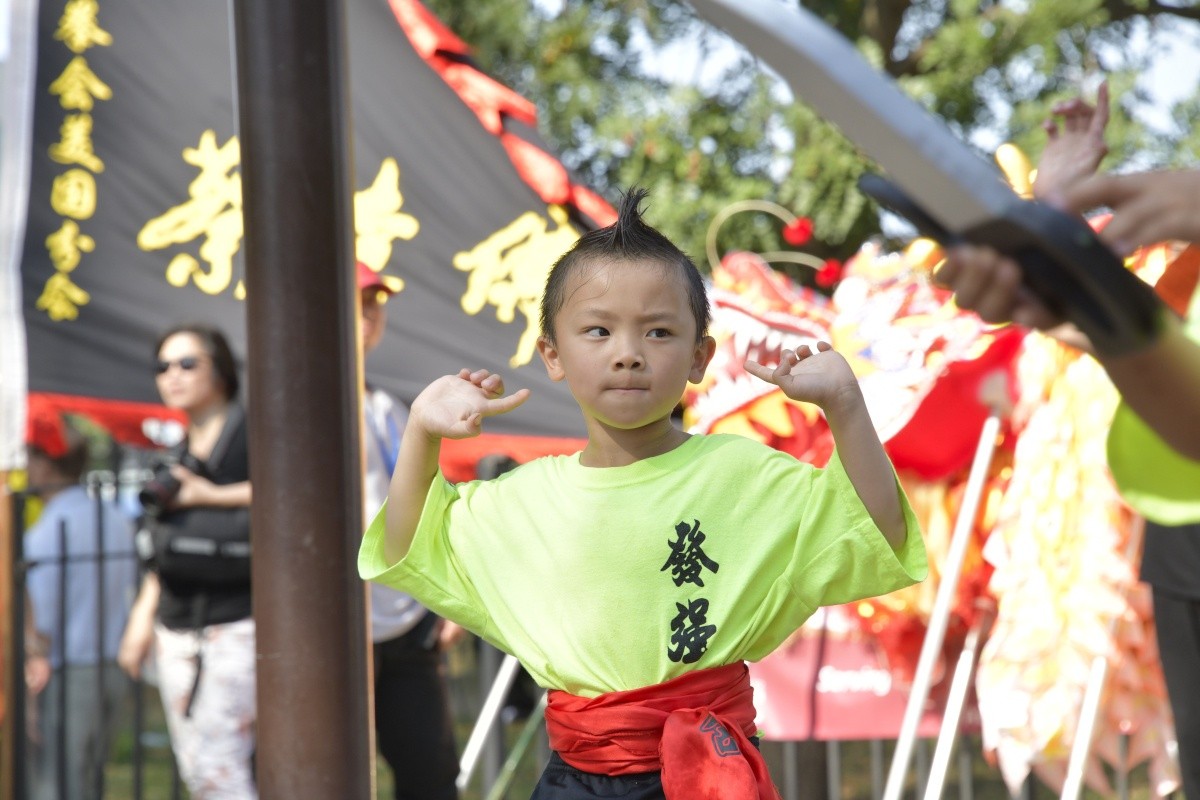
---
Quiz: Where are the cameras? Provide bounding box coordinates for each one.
[136,457,206,516]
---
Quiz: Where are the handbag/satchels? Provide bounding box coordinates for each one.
[147,518,257,593]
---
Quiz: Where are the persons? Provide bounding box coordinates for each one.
[938,83,1200,800]
[9,414,142,800]
[355,188,928,800]
[356,259,467,799]
[116,322,262,800]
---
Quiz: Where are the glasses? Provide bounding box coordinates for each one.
[154,356,201,374]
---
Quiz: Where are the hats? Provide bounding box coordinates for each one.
[357,258,399,297]
